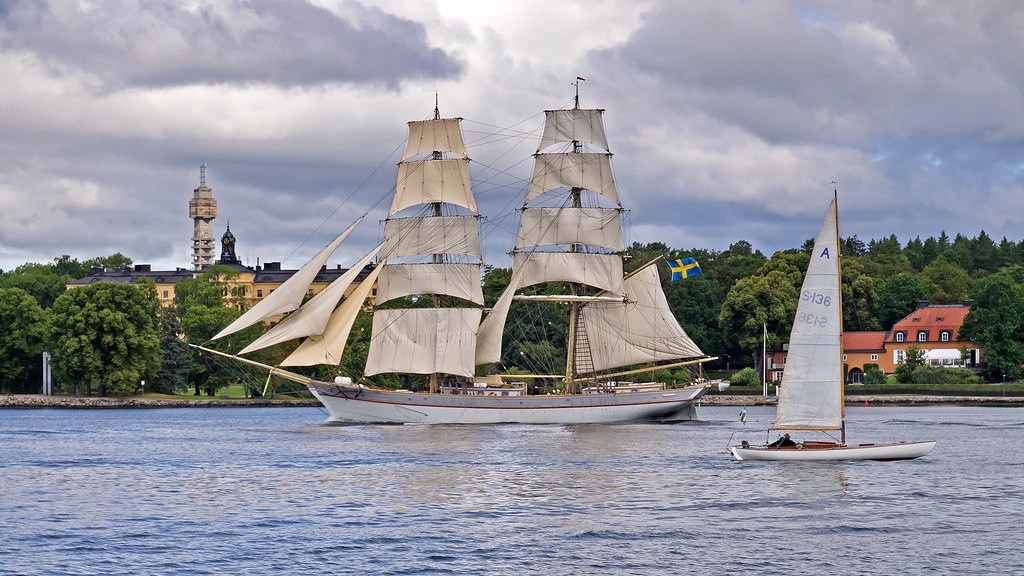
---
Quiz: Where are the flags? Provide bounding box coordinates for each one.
[739,405,747,424]
[666,257,702,281]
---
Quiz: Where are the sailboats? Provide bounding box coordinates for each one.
[186,75,730,426]
[725,179,938,464]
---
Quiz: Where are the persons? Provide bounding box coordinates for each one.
[779,434,797,446]
[532,386,540,395]
